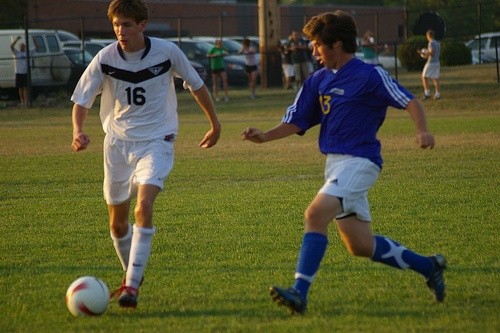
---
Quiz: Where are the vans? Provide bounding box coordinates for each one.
[0,29,82,101]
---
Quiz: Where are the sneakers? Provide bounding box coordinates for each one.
[269,285,307,314]
[110,287,138,307]
[427,255,447,302]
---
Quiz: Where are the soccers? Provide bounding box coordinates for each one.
[65,276,109,317]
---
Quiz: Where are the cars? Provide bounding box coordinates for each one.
[465,32,500,64]
[62,35,293,89]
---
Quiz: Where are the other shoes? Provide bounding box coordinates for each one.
[434,95,440,100]
[423,95,430,101]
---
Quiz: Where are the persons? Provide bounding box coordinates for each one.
[277,30,324,92]
[10,35,35,109]
[360,31,377,64]
[241,10,446,315]
[417,29,441,100]
[238,39,258,101]
[207,39,230,102]
[71,0,221,308]
